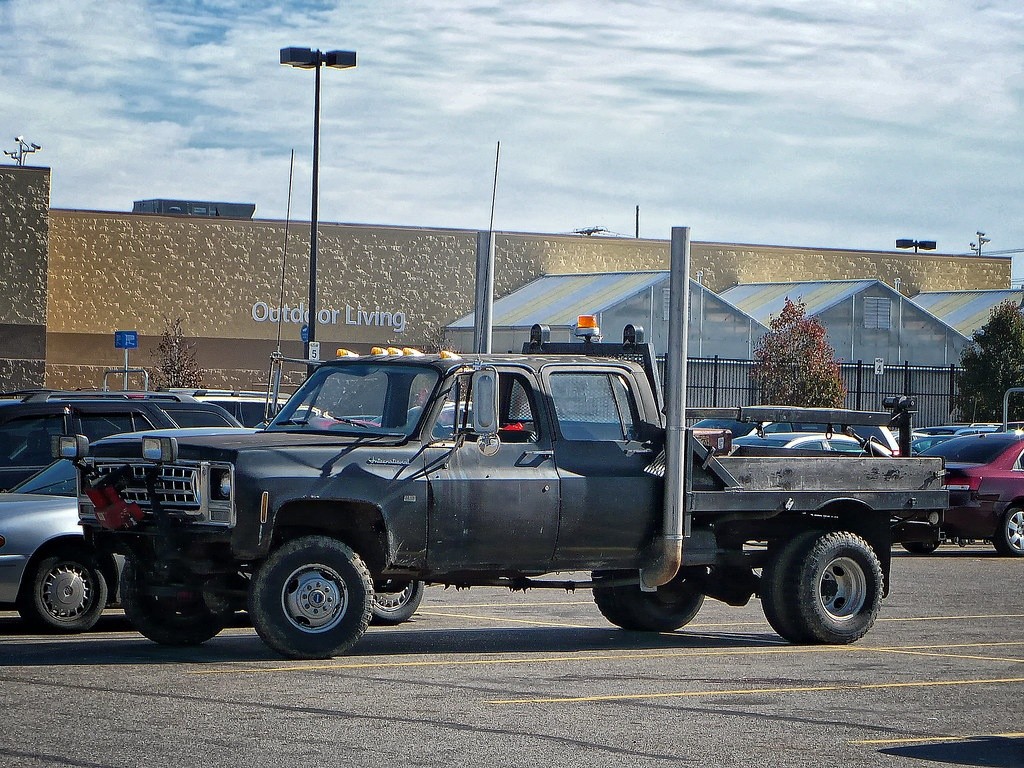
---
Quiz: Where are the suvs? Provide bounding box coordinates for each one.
[369,401,476,432]
[181,388,321,428]
[0,390,249,497]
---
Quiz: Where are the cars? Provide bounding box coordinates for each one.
[0,427,430,635]
[686,404,1024,559]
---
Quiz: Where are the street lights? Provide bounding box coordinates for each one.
[279,44,358,376]
[895,239,938,255]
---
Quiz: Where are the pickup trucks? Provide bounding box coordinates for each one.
[67,224,948,664]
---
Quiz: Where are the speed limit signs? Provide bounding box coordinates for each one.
[308,342,321,364]
